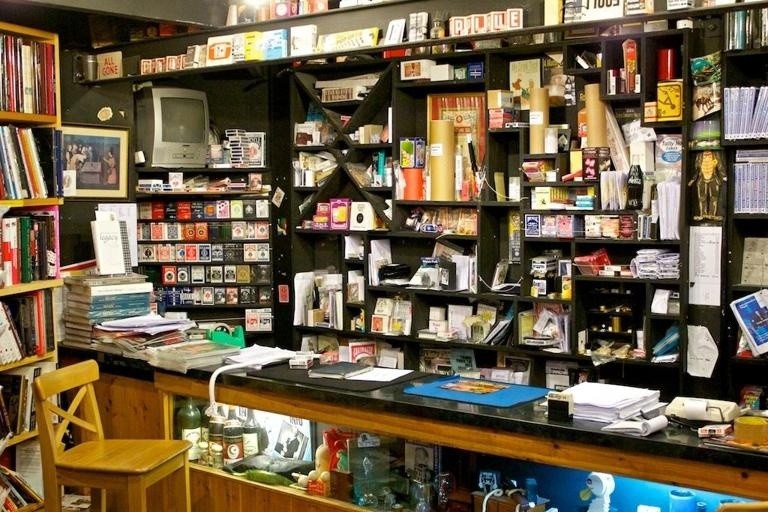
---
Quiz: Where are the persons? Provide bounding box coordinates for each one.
[62,142,117,186]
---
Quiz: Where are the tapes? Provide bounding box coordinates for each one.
[210,322,232,336]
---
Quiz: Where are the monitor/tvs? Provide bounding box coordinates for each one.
[134,87,210,169]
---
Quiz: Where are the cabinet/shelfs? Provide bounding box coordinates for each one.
[279,19,696,400]
[59,326,767,510]
[1,21,72,512]
[133,163,272,198]
[721,0,768,414]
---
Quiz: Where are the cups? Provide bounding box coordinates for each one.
[656,48,675,81]
[80,54,97,81]
[687,120,721,150]
[404,168,425,200]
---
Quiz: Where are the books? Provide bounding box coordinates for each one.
[62,268,153,348]
[2,34,64,512]
[728,288,768,359]
[601,414,670,437]
[305,361,370,380]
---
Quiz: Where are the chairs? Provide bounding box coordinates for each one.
[29,356,195,511]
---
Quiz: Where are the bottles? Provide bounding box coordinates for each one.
[430,16,445,38]
[315,433,330,474]
[386,161,393,188]
[175,397,263,468]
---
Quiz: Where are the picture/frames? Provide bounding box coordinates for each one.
[58,122,134,203]
